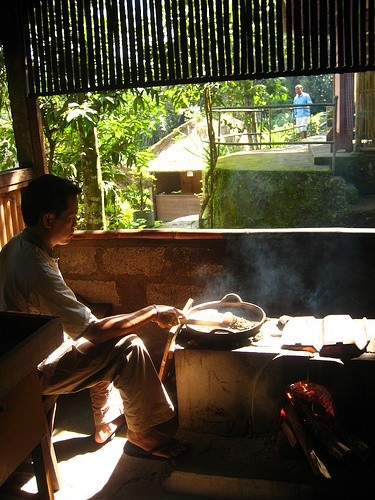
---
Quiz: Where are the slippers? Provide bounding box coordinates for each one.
[124,437,192,461]
[93,408,126,446]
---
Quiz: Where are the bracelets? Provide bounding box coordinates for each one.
[151,303,159,323]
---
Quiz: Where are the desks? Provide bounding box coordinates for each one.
[0,310,64,500]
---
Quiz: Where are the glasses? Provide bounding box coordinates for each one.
[70,213,81,220]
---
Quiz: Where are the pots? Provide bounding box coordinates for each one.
[183,293,267,346]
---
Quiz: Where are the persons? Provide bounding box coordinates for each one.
[292,84,313,140]
[1,174,193,462]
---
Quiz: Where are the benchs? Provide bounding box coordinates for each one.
[44,303,115,441]
